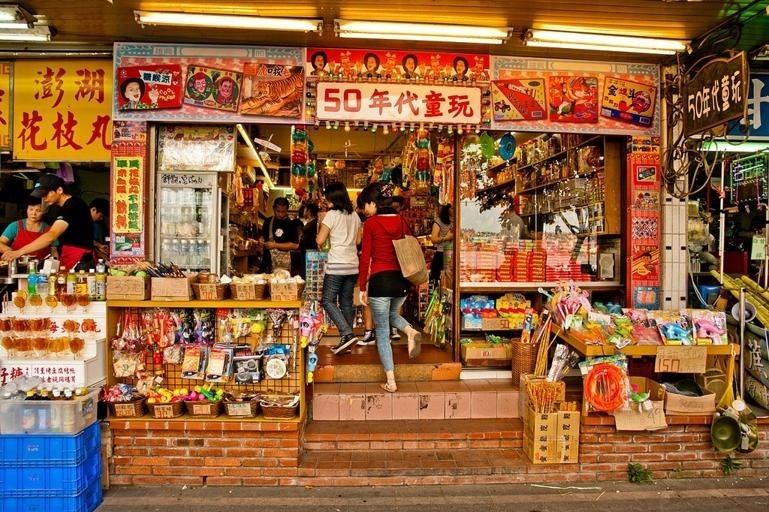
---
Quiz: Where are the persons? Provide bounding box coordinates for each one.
[214,77,237,104]
[312,182,402,354]
[256,196,322,281]
[0,174,99,274]
[354,180,423,395]
[634,189,658,275]
[499,194,524,237]
[0,193,61,261]
[525,210,553,240]
[429,204,458,283]
[86,198,110,256]
[307,49,491,83]
[188,71,212,99]
[120,75,161,110]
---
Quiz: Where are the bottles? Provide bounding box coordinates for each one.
[24,258,108,301]
[21,385,93,433]
[159,239,214,268]
[160,189,212,238]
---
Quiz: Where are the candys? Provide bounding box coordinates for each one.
[0,291,95,358]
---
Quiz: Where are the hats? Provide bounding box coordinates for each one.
[30,175,63,197]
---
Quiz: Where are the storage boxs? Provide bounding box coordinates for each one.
[521,402,580,463]
[107,271,199,301]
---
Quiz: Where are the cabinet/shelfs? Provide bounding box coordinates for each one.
[477,131,622,236]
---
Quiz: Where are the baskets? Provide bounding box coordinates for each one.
[191,283,305,300]
[511,338,540,391]
[111,398,299,418]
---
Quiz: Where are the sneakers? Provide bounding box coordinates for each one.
[331,327,398,354]
[380,382,397,392]
[408,330,421,357]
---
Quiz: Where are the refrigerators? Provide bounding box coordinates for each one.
[156,179,228,279]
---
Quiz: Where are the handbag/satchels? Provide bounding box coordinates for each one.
[392,235,427,285]
[270,249,292,270]
